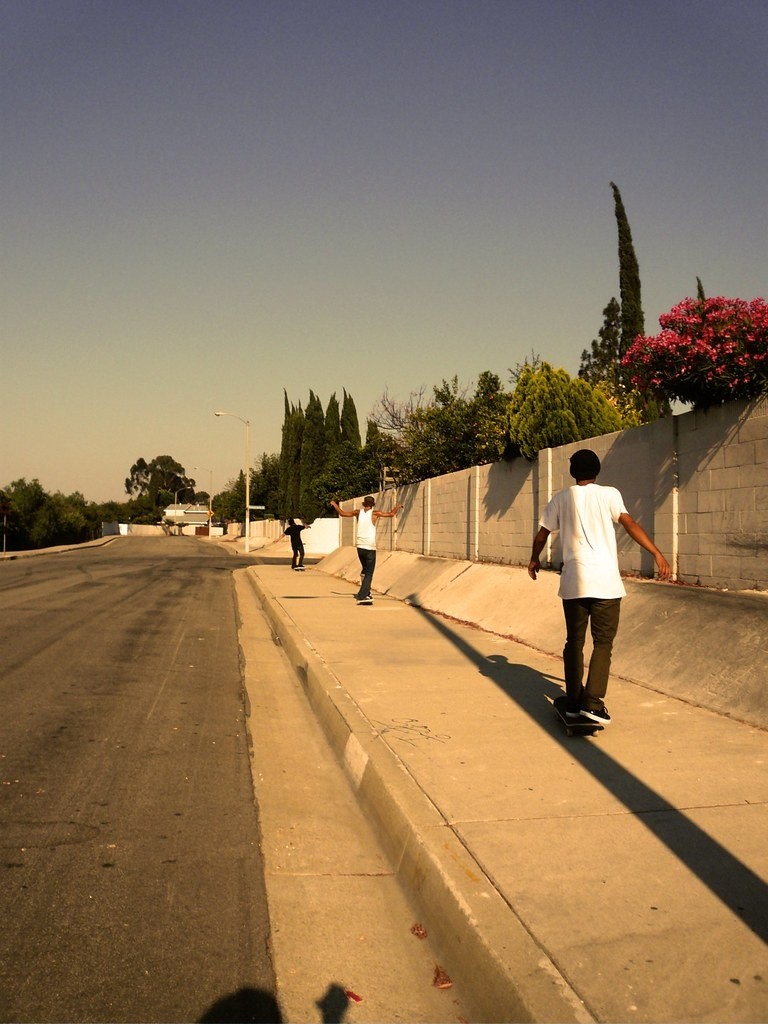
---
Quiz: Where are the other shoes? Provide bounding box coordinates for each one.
[368,593,374,599]
[363,596,371,600]
[297,565,305,569]
[292,565,298,569]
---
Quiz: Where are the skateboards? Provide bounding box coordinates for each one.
[553,695,605,737]
[356,596,373,605]
[293,567,305,571]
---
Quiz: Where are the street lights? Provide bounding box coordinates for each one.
[174,486,192,536]
[194,467,212,538]
[215,412,250,553]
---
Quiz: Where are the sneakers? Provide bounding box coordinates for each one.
[579,706,612,724]
[564,698,579,718]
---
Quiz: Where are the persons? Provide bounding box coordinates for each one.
[272,519,311,569]
[330,496,404,601]
[527,449,672,724]
